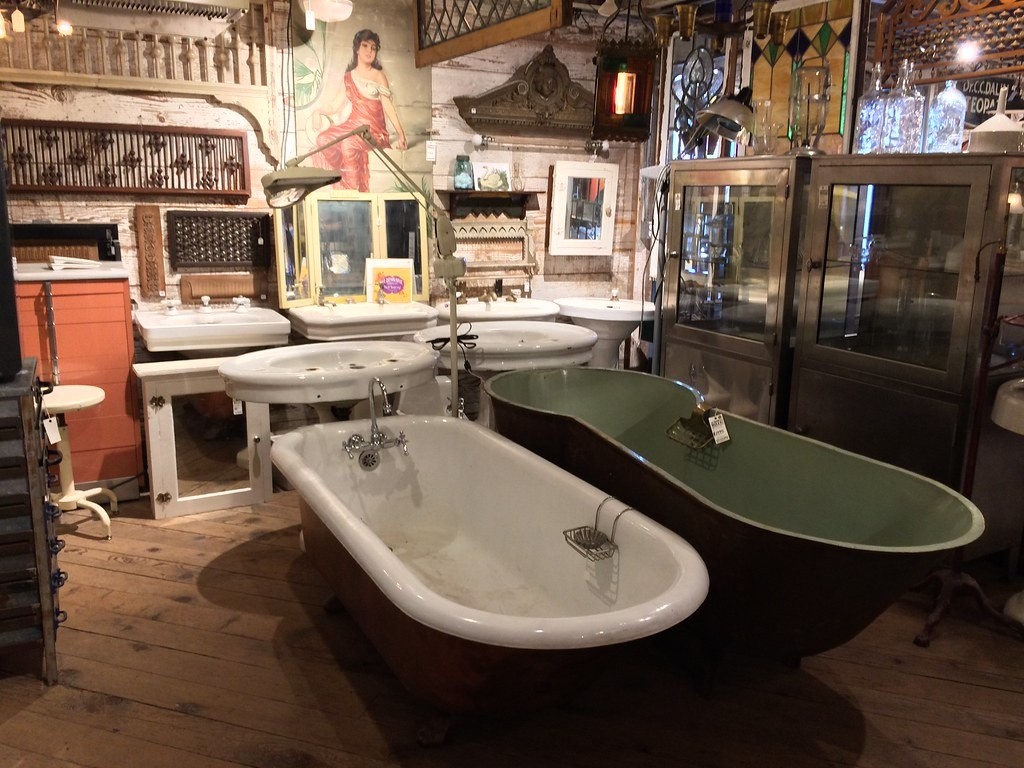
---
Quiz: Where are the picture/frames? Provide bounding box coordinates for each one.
[472,162,512,191]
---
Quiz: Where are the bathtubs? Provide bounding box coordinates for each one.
[480,365,986,660]
[268,413,710,722]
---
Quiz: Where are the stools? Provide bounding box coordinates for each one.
[33,385,117,540]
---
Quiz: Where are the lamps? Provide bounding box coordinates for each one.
[261,124,468,417]
[592,0,658,144]
[652,87,758,374]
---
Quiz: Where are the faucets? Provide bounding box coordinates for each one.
[374,283,386,305]
[198,295,213,313]
[610,289,620,302]
[478,289,498,302]
[317,284,337,307]
[341,376,410,460]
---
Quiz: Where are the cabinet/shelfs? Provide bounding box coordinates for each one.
[787,151,1024,580]
[434,184,547,279]
[656,158,798,432]
[13,261,146,498]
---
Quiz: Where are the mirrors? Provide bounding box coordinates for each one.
[549,161,619,256]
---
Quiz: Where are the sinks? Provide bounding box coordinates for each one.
[218,340,441,404]
[990,377,1024,435]
[435,295,561,326]
[135,307,292,352]
[413,320,599,372]
[33,384,106,415]
[552,296,655,339]
[288,301,439,341]
